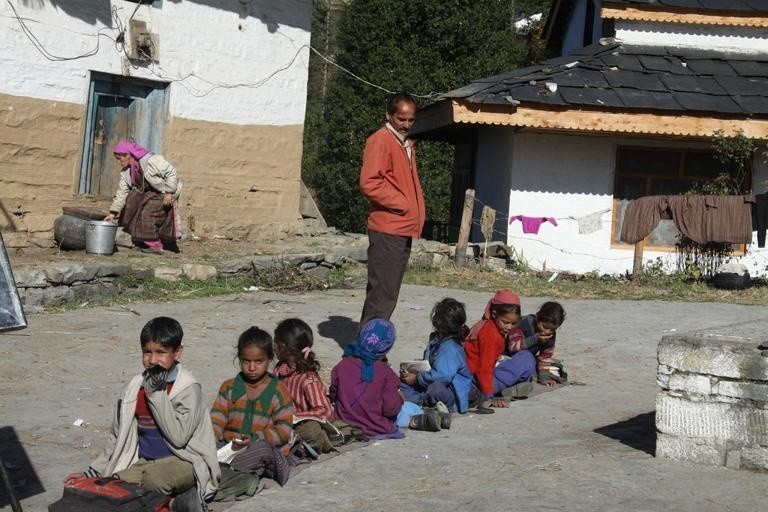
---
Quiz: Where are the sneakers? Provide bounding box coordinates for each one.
[141,248,164,255]
[171,486,204,512]
[502,381,533,397]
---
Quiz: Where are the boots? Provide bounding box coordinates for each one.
[410,401,450,432]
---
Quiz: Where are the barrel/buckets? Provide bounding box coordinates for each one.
[84,219,118,255]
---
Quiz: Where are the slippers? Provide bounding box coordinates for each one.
[297,440,319,459]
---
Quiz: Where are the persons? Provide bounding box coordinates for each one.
[395,401,452,432]
[516,302,566,388]
[465,290,535,407]
[103,141,182,255]
[64,317,219,512]
[208,327,294,501]
[271,318,332,461]
[355,92,426,363]
[399,296,476,412]
[326,317,402,441]
